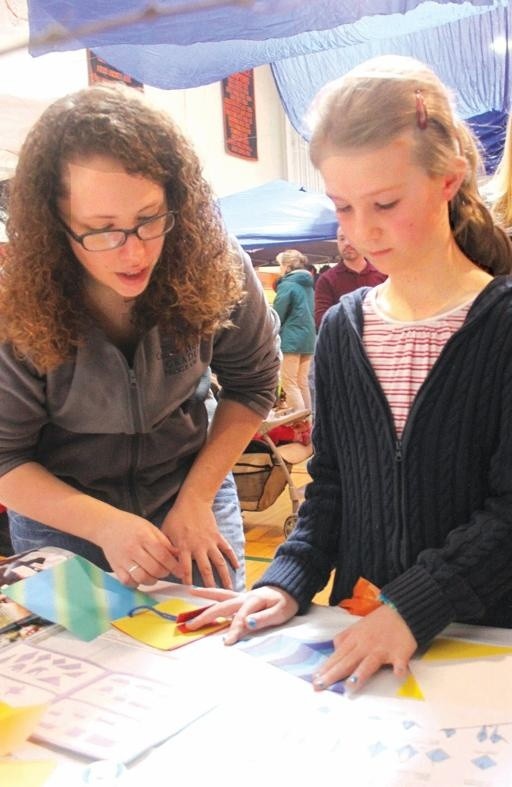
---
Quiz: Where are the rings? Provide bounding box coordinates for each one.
[128,563,140,573]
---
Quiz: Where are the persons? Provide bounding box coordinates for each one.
[272,250,318,426]
[0,83,283,595]
[307,265,318,288]
[184,56,511,694]
[313,224,386,333]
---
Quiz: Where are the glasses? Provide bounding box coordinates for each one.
[48,202,177,252]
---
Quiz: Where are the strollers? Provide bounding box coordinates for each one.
[208,382,315,538]
